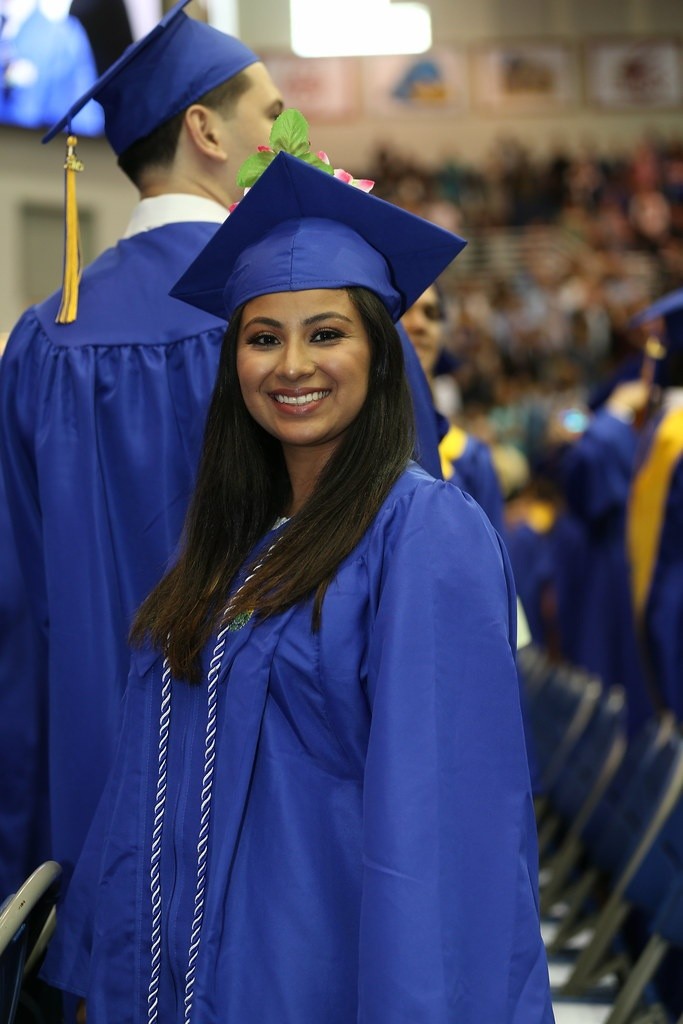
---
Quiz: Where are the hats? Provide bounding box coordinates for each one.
[39,0,257,157]
[169,153,469,321]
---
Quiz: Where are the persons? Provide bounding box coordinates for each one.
[366,129,682,445]
[1,0,444,1023]
[562,334,663,681]
[626,288,683,733]
[396,279,501,540]
[35,154,557,1024]
